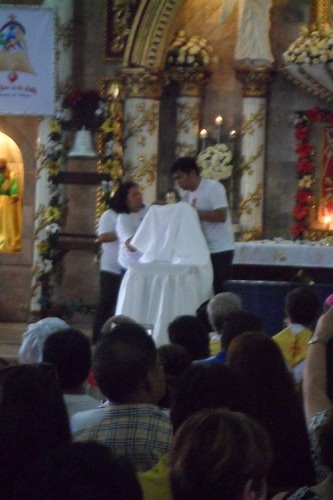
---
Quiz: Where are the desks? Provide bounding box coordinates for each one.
[232,238,333,281]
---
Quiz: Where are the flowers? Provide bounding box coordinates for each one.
[164,30,219,88]
[30,88,124,319]
[281,23,333,65]
[195,143,233,181]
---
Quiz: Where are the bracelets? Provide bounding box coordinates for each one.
[307,338,328,345]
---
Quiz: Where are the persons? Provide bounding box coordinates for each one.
[92,155,235,348]
[1,286,333,500]
